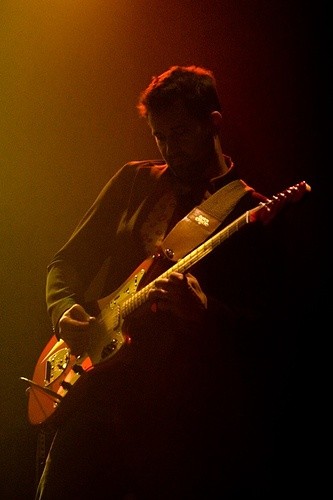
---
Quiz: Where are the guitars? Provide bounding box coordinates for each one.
[28,180,311,433]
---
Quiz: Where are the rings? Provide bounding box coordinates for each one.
[159,289,168,298]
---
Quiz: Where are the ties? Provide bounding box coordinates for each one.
[135,183,191,261]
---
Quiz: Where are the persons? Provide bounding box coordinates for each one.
[34,66,290,500]
